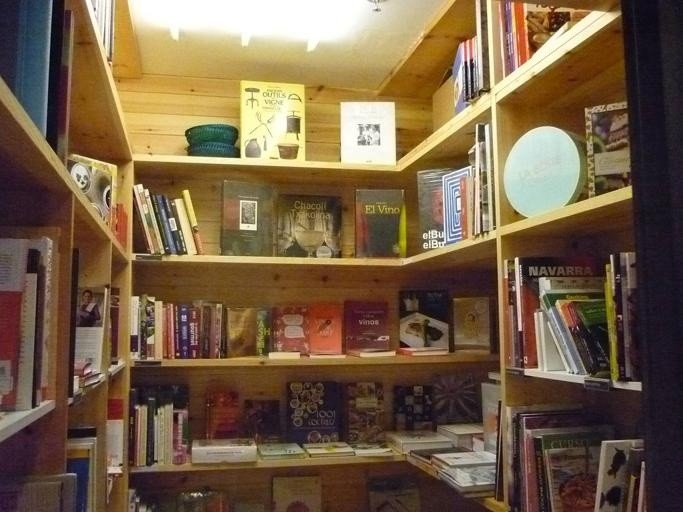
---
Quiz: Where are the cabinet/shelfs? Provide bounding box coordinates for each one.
[2,0,135,511]
[135,152,403,512]
[381,3,672,511]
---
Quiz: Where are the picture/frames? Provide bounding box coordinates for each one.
[584,101,634,197]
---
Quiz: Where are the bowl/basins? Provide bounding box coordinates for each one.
[184,123,240,157]
